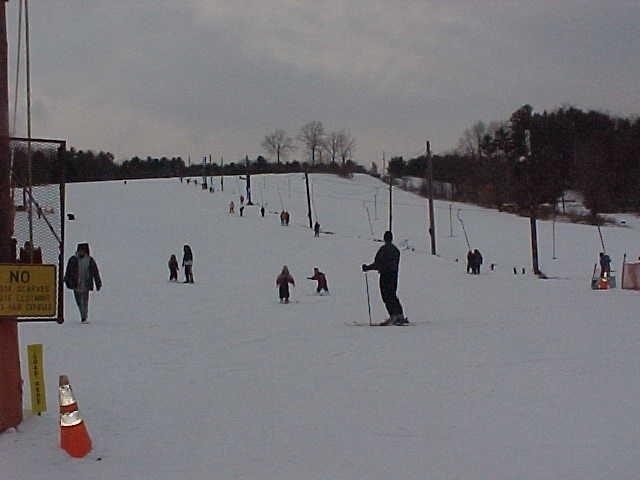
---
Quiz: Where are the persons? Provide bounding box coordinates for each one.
[228,195,289,226]
[361,231,408,325]
[276,264,296,303]
[181,245,197,284]
[168,254,181,281]
[66,242,102,321]
[464,249,483,274]
[597,251,612,278]
[313,221,320,237]
[305,267,329,297]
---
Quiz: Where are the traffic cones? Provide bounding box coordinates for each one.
[57,373,93,459]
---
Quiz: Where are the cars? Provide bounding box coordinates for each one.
[538,199,589,215]
